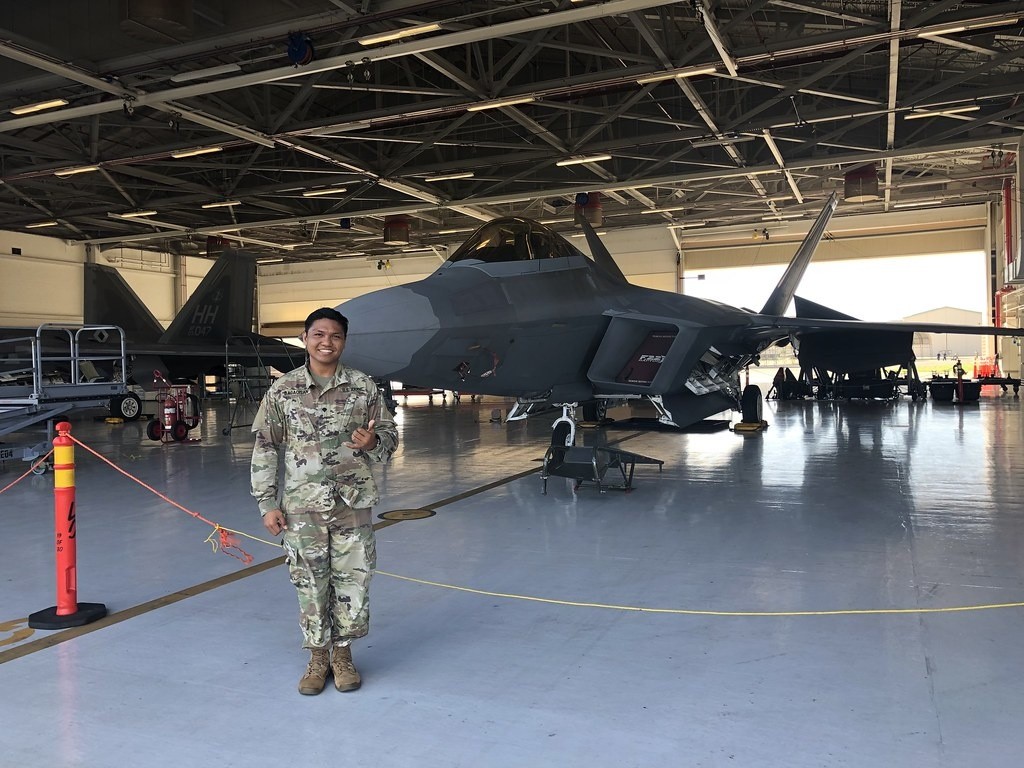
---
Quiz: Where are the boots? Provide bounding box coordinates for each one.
[298,648,330,696]
[331,644,360,692]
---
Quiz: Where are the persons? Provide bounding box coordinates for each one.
[943,351,947,361]
[249,307,399,694]
[937,352,940,360]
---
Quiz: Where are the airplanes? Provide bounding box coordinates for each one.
[18,251,306,423]
[330,190,1024,461]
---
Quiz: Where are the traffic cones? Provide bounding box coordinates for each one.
[29,422,106,631]
